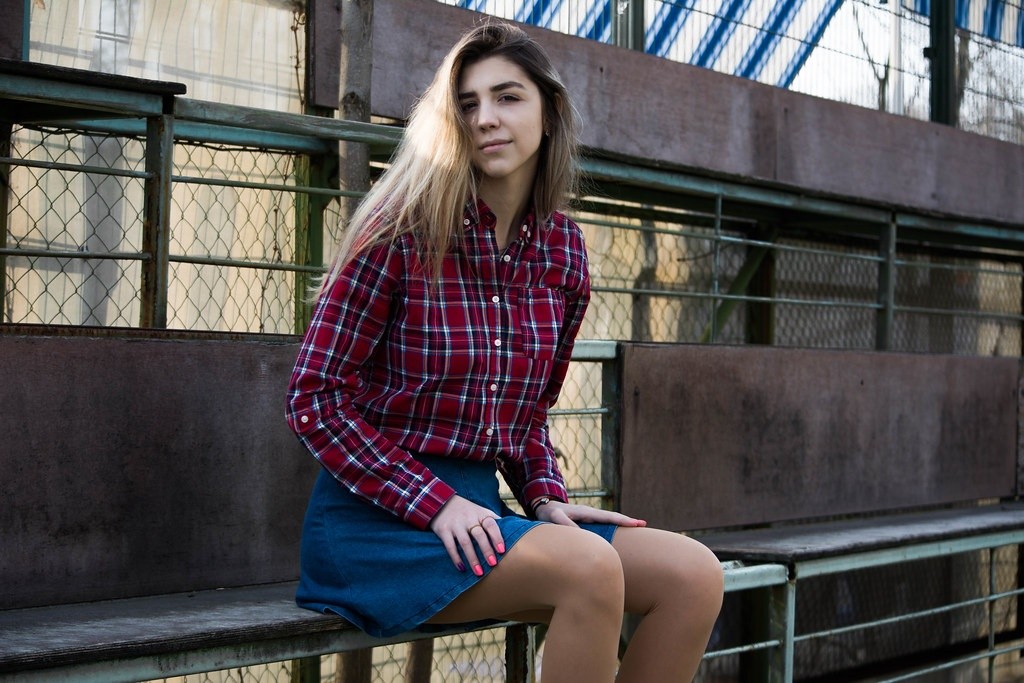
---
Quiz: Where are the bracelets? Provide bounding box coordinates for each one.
[533,498,565,521]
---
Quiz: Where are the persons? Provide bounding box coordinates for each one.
[284,22,723,683]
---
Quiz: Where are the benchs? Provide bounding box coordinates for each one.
[0,325,1024,683]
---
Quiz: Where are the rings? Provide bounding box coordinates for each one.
[469,524,481,533]
[480,516,494,527]
